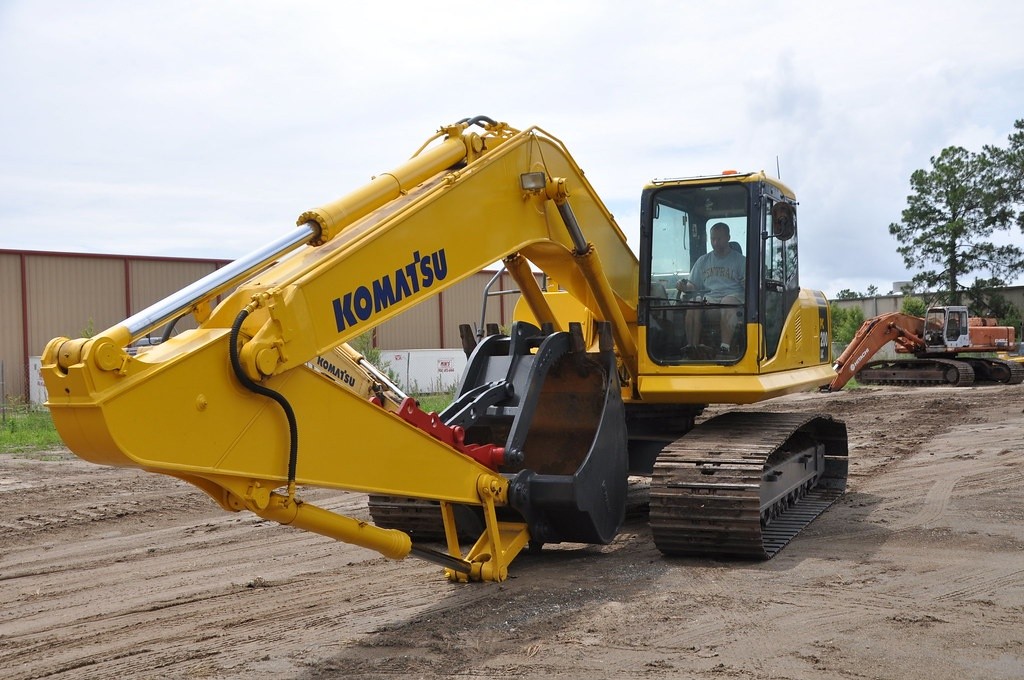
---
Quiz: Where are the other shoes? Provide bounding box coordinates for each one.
[718,347,729,355]
[680,346,695,351]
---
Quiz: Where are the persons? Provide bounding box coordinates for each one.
[677,221,747,360]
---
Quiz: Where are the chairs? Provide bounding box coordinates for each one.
[703,241,744,325]
[943,318,958,338]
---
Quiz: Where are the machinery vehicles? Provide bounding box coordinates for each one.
[41,113,849,585]
[820,303,1023,387]
